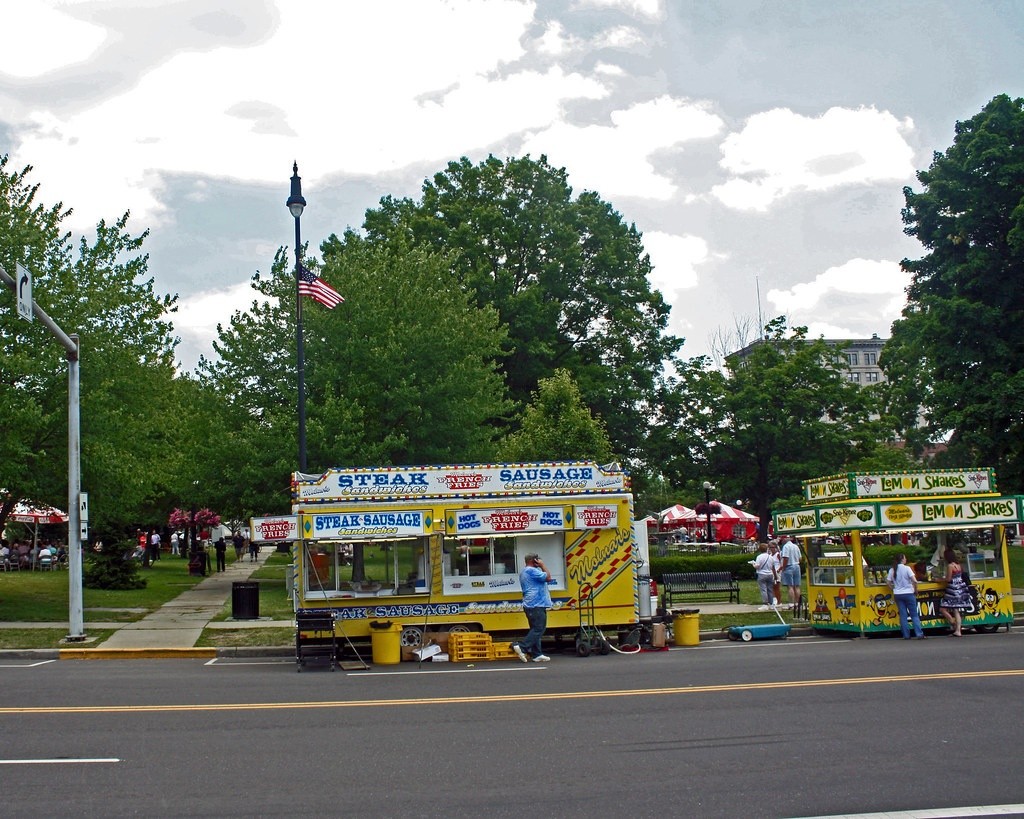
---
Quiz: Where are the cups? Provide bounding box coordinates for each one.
[454,569,459,576]
[927,571,932,581]
[845,576,854,584]
[993,571,998,577]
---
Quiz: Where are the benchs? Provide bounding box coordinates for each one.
[662,572,740,605]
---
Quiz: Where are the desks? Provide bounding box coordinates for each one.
[338,552,345,566]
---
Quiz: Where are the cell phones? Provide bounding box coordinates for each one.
[533,560,538,564]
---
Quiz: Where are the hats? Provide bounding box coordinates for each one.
[525,553,540,563]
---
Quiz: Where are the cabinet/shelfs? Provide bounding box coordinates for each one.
[294,613,338,671]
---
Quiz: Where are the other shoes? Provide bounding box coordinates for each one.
[905,637,911,640]
[918,636,927,640]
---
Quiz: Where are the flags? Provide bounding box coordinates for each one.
[298,263,345,309]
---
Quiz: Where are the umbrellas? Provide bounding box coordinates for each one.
[0,488,15,514]
[0,498,69,568]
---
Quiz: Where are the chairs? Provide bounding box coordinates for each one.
[966,554,987,579]
[0,553,68,572]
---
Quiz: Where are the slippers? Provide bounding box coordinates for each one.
[948,633,962,637]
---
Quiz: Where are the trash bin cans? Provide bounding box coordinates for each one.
[232,581,259,619]
[671,609,701,646]
[369,623,403,665]
[189,551,207,576]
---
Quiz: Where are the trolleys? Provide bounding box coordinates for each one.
[574,581,608,657]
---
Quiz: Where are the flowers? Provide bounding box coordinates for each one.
[168,507,220,529]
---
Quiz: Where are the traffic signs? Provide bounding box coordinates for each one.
[16,261,33,323]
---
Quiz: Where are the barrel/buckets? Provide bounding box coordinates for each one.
[487,563,506,574]
[650,596,659,616]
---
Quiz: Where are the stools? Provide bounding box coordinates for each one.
[793,593,808,621]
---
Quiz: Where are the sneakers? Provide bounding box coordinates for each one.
[782,603,798,610]
[513,645,527,662]
[532,654,550,662]
[758,603,782,611]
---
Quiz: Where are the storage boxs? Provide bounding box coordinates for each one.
[401,632,527,663]
[652,623,666,647]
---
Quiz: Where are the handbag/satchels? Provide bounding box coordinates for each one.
[961,571,972,586]
[799,562,806,574]
[753,572,758,581]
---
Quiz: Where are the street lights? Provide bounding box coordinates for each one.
[285,161,307,474]
[703,481,712,542]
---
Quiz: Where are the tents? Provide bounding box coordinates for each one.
[672,499,760,542]
[641,503,697,533]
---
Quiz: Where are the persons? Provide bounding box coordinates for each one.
[0,528,259,573]
[931,548,971,637]
[886,553,927,640]
[671,525,708,543]
[459,542,472,554]
[337,542,354,565]
[929,544,954,571]
[861,530,924,546]
[747,533,802,610]
[755,522,761,536]
[513,553,551,664]
